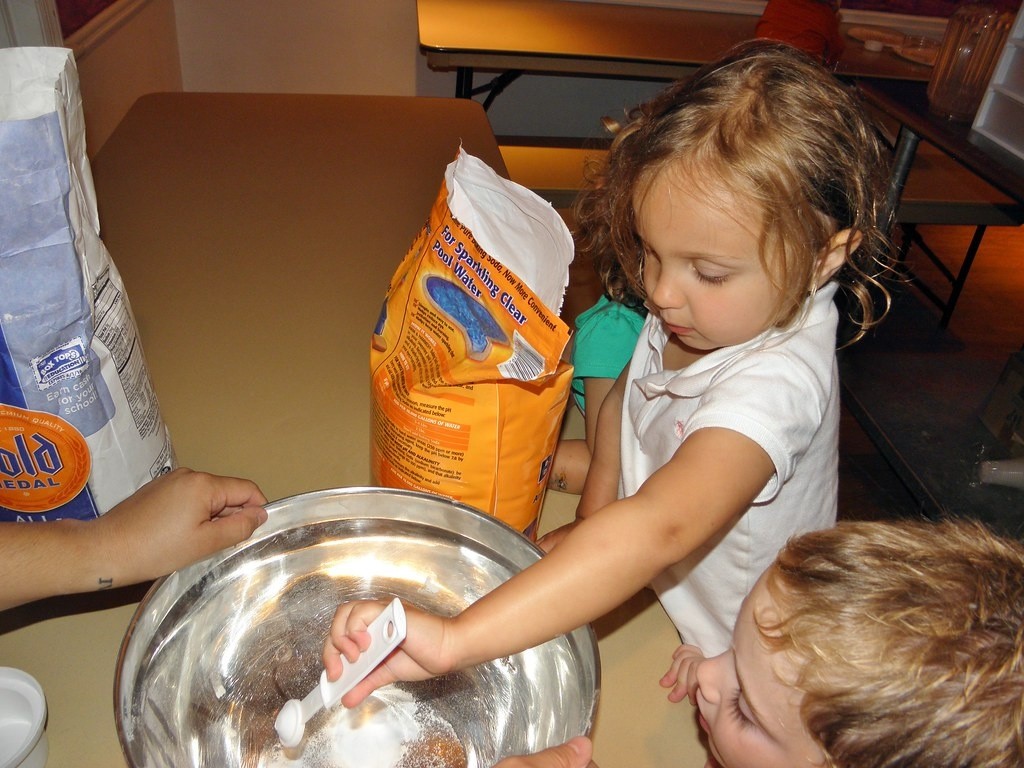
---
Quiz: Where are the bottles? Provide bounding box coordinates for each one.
[926,0,1018,127]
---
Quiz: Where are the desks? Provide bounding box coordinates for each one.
[842,80,1024,541]
[0,91,719,768]
[416,0,944,112]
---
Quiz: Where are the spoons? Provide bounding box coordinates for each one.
[272,596,407,749]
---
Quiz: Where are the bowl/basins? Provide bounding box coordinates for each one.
[902,35,937,63]
[112,484,602,768]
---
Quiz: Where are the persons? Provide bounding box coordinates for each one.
[658,518,1024,768]
[322,37,911,712]
[0,466,600,768]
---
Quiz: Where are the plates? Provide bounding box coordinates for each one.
[845,24,940,68]
[0,664,48,768]
